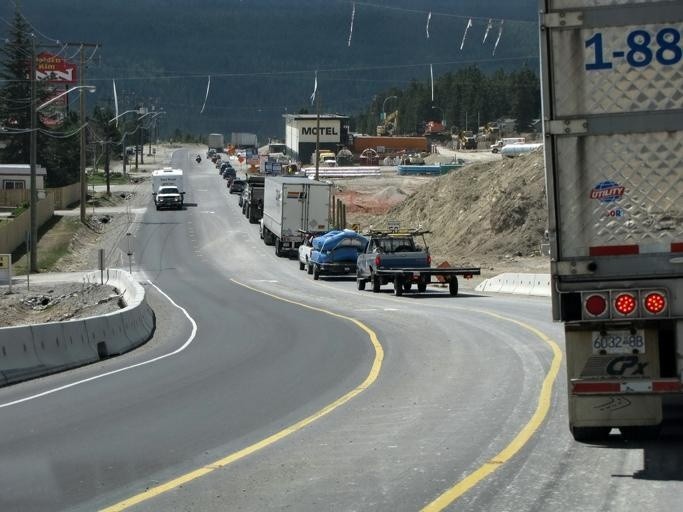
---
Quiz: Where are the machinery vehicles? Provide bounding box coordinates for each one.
[456,130,478,150]
[377,110,400,136]
[424,120,450,142]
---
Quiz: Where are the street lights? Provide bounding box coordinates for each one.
[135,111,166,170]
[28,35,96,273]
[432,106,446,129]
[105,111,145,195]
[384,95,398,135]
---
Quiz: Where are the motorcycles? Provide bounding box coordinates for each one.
[196,157,201,163]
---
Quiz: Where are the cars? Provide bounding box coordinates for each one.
[207,149,245,193]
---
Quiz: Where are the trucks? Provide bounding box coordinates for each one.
[152,167,185,209]
[299,226,368,280]
[314,150,337,167]
[209,133,225,152]
[355,228,480,296]
[239,175,264,223]
[490,137,525,153]
[258,175,332,257]
[535,1,683,439]
